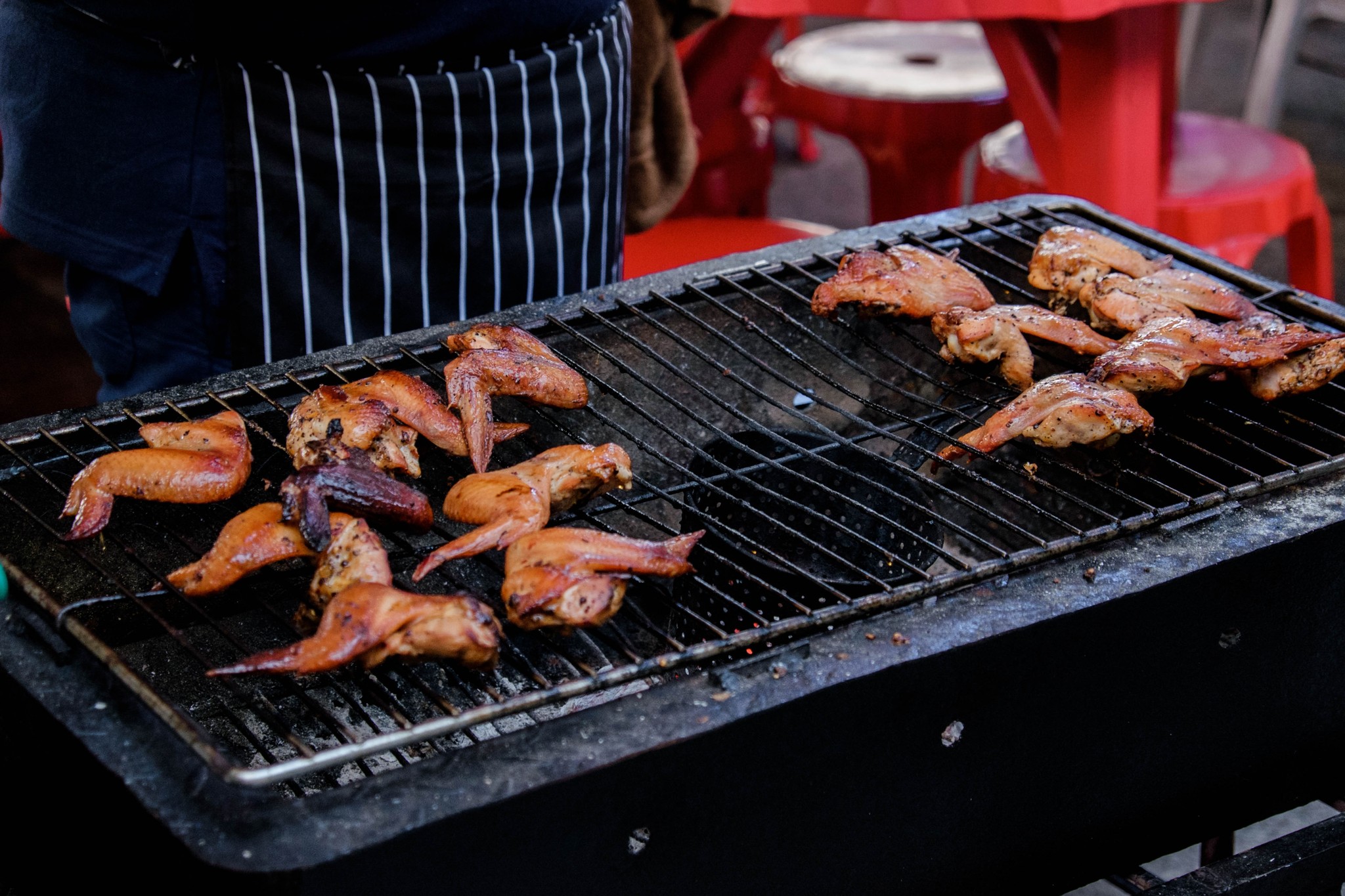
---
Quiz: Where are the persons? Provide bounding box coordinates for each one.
[0,0,699,404]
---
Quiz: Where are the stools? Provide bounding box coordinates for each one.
[956,110,1333,300]
[774,16,1012,218]
